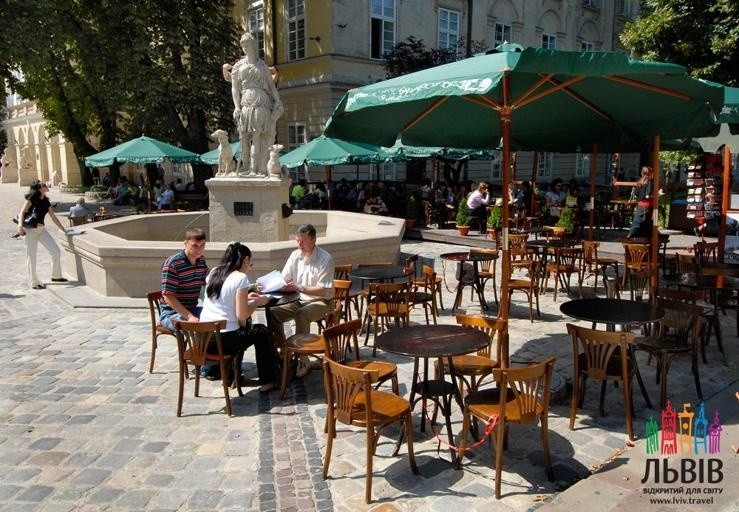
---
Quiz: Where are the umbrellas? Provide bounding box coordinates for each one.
[323,39,739,451]
[631,80,739,353]
[279,136,377,181]
[84,135,200,214]
[377,136,495,177]
[200,142,285,166]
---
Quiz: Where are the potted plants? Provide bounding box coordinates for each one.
[486,204,502,241]
[404,196,417,228]
[454,199,471,236]
[551,208,575,236]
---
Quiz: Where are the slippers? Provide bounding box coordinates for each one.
[259,379,282,390]
[224,374,244,386]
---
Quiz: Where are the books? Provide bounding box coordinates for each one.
[685,153,722,226]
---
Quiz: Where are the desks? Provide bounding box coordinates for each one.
[439,252,499,312]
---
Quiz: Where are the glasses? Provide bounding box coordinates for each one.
[42,184,46,188]
[249,259,253,268]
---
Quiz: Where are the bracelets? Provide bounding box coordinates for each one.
[299,285,305,292]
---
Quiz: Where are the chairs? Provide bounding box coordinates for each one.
[575,184,657,232]
[625,234,738,276]
[320,355,421,504]
[331,263,361,321]
[633,299,704,413]
[170,318,246,419]
[145,288,191,378]
[364,282,413,358]
[404,265,437,325]
[462,200,482,234]
[70,182,208,226]
[667,283,726,361]
[403,254,445,312]
[278,304,344,401]
[312,279,353,353]
[322,319,401,434]
[470,248,500,308]
[502,235,624,320]
[357,263,395,289]
[629,273,654,305]
[292,183,403,212]
[432,313,507,437]
[506,191,549,234]
[566,322,639,442]
[455,357,557,500]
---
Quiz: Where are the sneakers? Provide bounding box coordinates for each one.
[52,278,67,282]
[279,350,294,366]
[207,373,222,380]
[32,284,45,289]
[297,359,311,377]
[192,366,206,378]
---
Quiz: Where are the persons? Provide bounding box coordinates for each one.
[567,178,581,196]
[94,164,183,209]
[272,223,335,379]
[290,179,467,227]
[467,182,490,234]
[160,228,221,379]
[627,166,668,243]
[198,241,282,392]
[18,179,73,289]
[546,178,567,207]
[519,181,533,216]
[231,33,284,178]
[70,198,92,226]
[507,183,524,210]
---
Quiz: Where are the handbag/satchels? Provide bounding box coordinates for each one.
[12,212,38,228]
